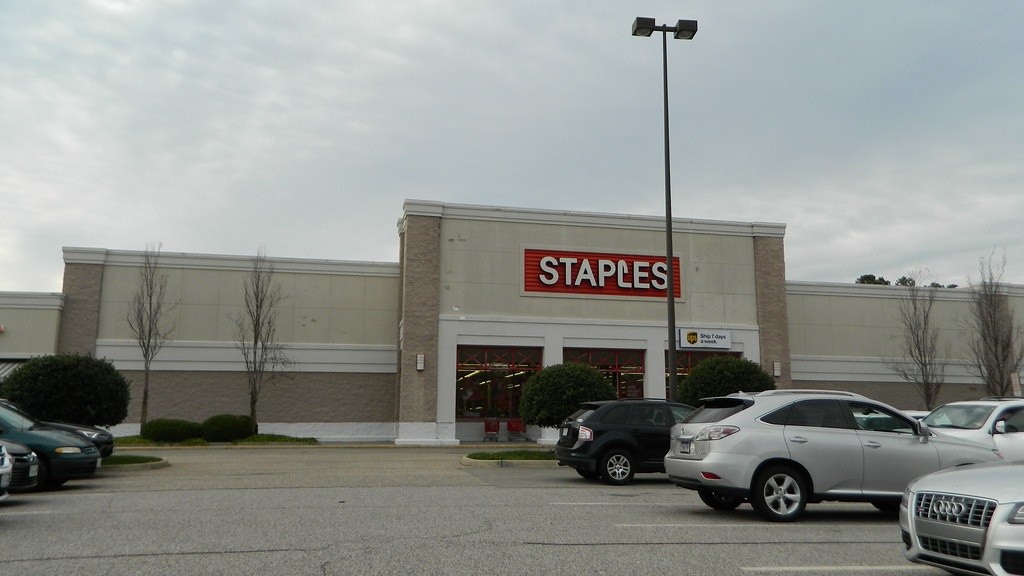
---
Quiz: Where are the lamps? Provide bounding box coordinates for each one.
[417,354,425,371]
[773,361,780,376]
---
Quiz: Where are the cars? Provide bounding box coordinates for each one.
[552,398,703,487]
[0,397,114,458]
[665,388,1005,523]
[889,400,1024,464]
[899,461,1024,576]
[0,439,46,498]
[0,402,103,489]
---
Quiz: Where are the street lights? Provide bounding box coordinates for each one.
[632,13,698,403]
